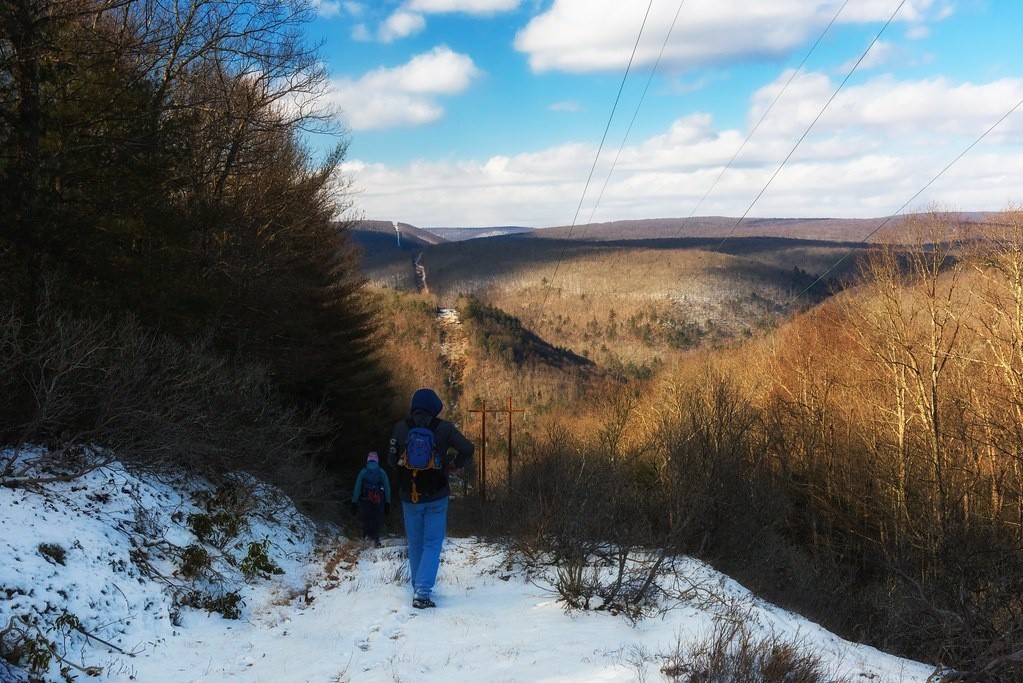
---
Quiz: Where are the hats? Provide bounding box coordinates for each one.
[368,452,379,463]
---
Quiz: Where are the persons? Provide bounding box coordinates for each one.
[386,389,475,609]
[350,451,391,548]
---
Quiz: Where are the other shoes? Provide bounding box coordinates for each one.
[412,599,435,609]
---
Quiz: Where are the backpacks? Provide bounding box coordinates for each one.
[364,467,385,509]
[402,419,442,470]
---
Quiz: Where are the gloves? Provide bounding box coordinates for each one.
[385,503,390,510]
[352,502,357,511]
